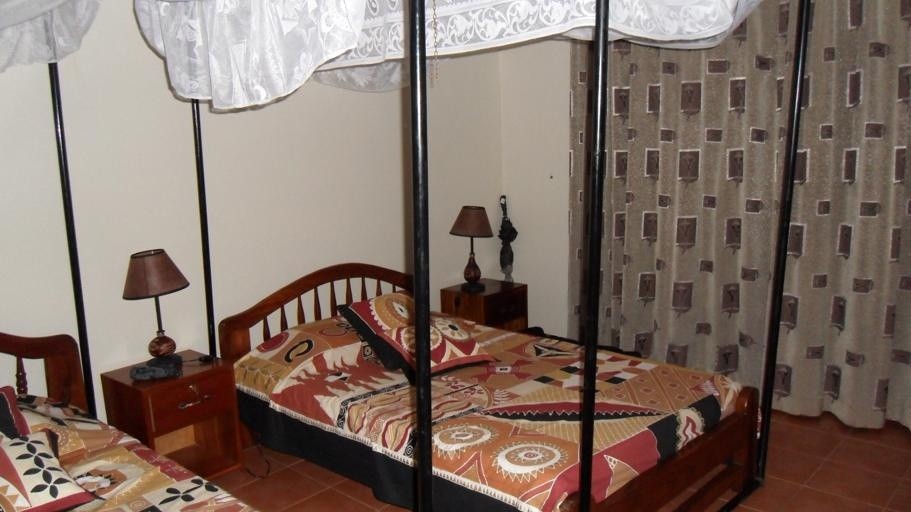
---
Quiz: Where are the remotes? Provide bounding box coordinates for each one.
[128,366,181,381]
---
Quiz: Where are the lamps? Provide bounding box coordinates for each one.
[450,205,494,294]
[122,249,191,359]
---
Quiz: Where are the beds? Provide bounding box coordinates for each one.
[1,332,256,512]
[219,262,760,511]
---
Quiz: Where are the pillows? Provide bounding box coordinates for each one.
[1,386,96,512]
[337,289,496,385]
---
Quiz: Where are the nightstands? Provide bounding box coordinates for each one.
[100,348,243,481]
[440,278,528,332]
